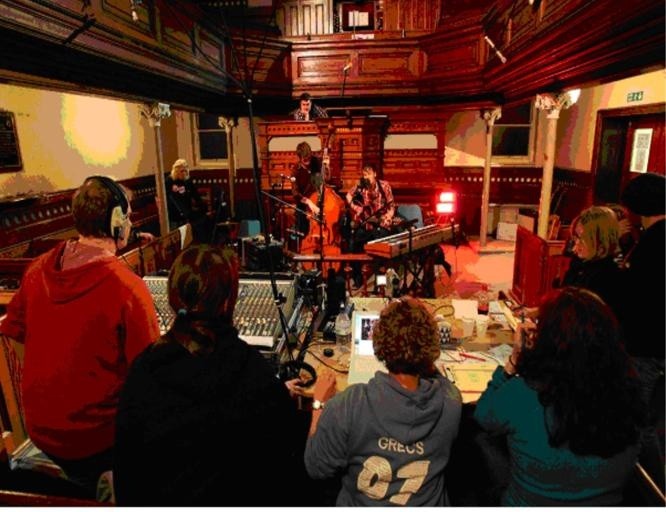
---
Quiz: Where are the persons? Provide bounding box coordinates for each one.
[295,93,329,122]
[301,294,464,506]
[166,156,220,248]
[558,173,666,494]
[291,140,332,248]
[0,173,163,501]
[345,164,402,293]
[112,245,308,506]
[456,285,666,507]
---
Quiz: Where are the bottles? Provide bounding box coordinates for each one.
[335,306,352,371]
[476,283,490,315]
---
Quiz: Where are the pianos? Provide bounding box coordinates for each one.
[364,224,460,258]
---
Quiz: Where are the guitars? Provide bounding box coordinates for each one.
[350,200,389,230]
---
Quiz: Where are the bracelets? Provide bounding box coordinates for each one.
[310,399,326,411]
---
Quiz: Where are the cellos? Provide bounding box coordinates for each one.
[301,148,345,280]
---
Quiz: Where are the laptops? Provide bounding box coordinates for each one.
[348,310,386,385]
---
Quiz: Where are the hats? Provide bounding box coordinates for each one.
[621,172,665,217]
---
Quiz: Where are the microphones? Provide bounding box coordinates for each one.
[279,172,298,184]
[343,61,354,73]
[484,35,509,67]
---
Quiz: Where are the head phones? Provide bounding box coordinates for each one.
[107,203,130,243]
[280,359,319,391]
